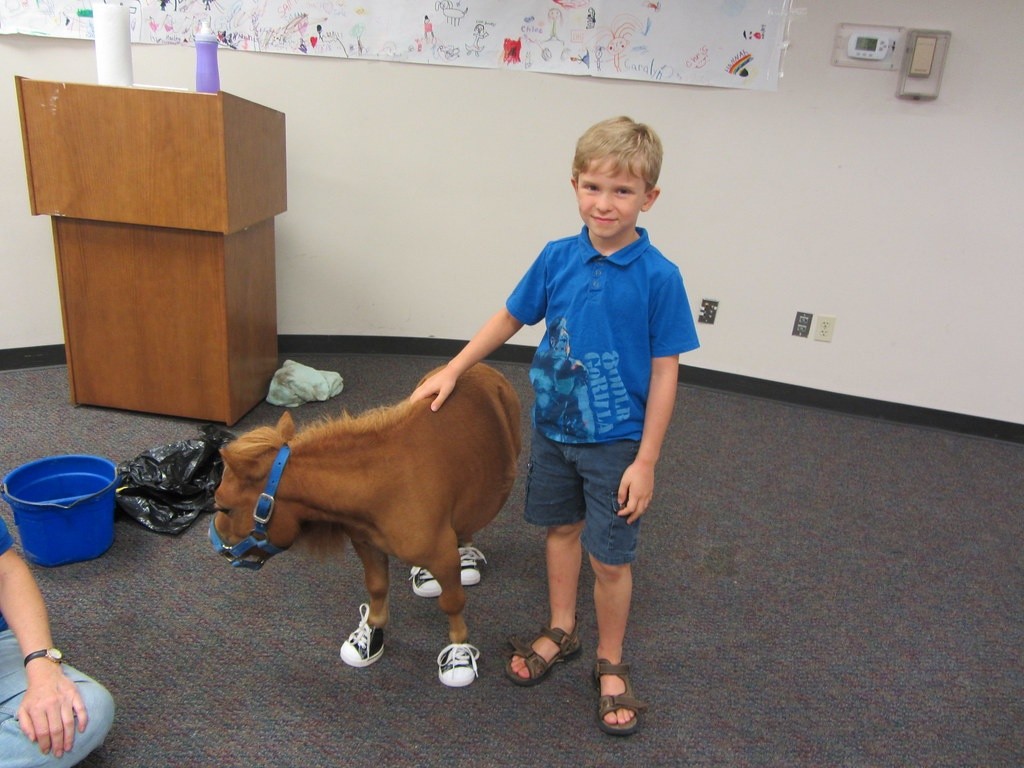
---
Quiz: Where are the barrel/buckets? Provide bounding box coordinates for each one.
[0,453,118,568]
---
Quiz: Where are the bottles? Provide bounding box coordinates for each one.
[195,20,221,93]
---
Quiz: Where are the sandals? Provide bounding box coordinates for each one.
[592,656,645,736]
[506,620,583,686]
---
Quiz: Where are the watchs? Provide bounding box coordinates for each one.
[24,647,62,668]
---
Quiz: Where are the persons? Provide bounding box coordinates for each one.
[410,117,700,735]
[0,516,114,768]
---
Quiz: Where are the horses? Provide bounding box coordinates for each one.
[209,360,522,686]
